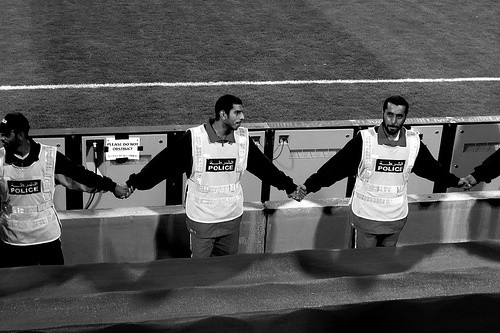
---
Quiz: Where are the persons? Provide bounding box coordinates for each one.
[122,94,306,258]
[464,148,500,190]
[293,95,471,249]
[0,112,129,268]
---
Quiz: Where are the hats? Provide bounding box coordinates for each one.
[0,112,30,133]
